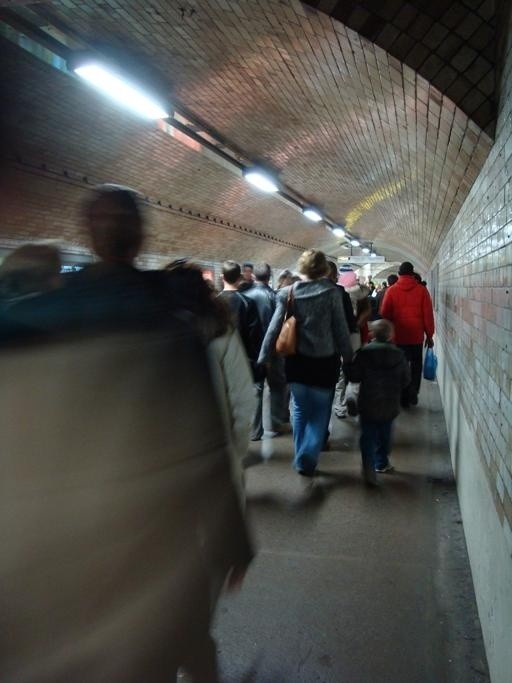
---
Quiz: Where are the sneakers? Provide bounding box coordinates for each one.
[375,463,393,473]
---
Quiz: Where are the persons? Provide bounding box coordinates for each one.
[215,249,434,492]
[1,183,251,682]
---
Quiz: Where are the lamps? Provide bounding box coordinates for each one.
[303,207,322,223]
[65,48,173,121]
[242,167,280,194]
[332,226,377,258]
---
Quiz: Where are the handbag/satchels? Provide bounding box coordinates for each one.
[424,348,437,380]
[274,285,297,357]
